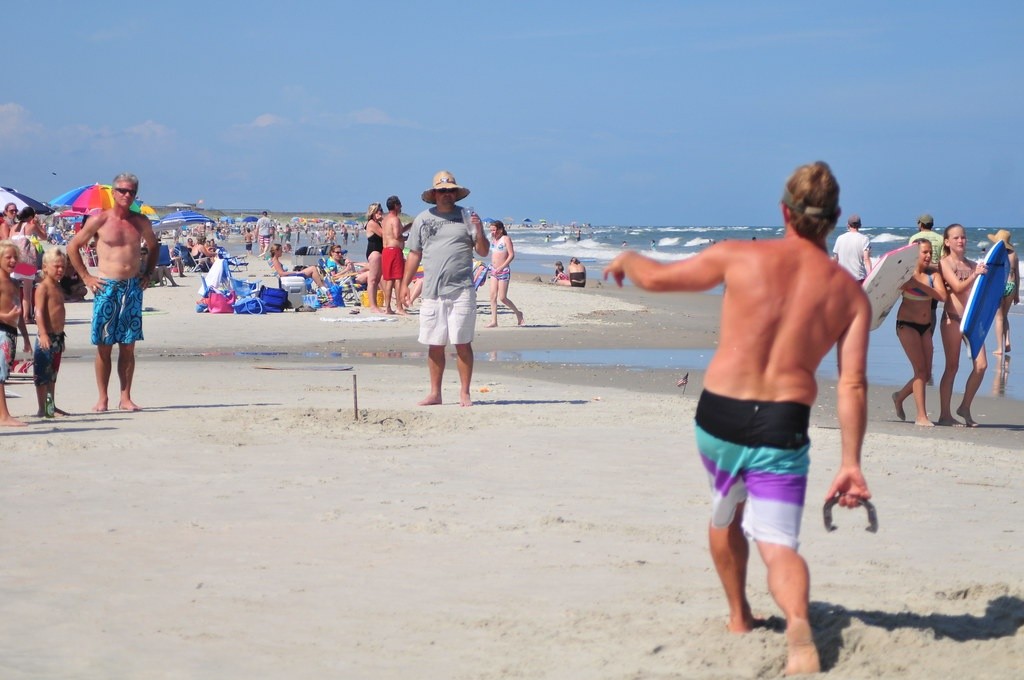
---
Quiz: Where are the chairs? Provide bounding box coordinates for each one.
[318,258,366,304]
[156,244,281,302]
[412,258,489,292]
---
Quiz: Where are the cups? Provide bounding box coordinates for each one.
[461,207,477,234]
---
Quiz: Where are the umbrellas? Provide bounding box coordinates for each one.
[0,186,56,215]
[155,207,221,239]
[48,183,155,217]
[242,216,258,222]
[153,209,216,230]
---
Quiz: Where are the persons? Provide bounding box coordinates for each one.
[601,161,869,676]
[400,170,490,406]
[188,211,280,265]
[66,174,159,411]
[0,202,71,426]
[831,215,1020,425]
[268,222,369,293]
[545,220,757,288]
[365,195,423,315]
[484,219,523,328]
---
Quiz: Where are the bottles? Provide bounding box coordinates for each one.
[303,295,315,308]
[45,392,54,418]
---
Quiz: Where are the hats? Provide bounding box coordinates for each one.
[421,171,470,204]
[916,214,934,225]
[848,215,860,224]
[988,229,1013,250]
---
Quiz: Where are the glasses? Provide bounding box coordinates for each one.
[435,189,457,193]
[114,188,136,196]
[378,208,382,212]
[397,202,401,205]
[8,209,18,213]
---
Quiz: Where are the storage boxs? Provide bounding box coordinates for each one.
[281,276,306,309]
[289,254,329,267]
[360,289,385,308]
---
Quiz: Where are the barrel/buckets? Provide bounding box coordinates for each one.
[360,290,384,308]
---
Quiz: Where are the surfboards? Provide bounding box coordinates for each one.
[960,240,1012,360]
[859,242,921,331]
